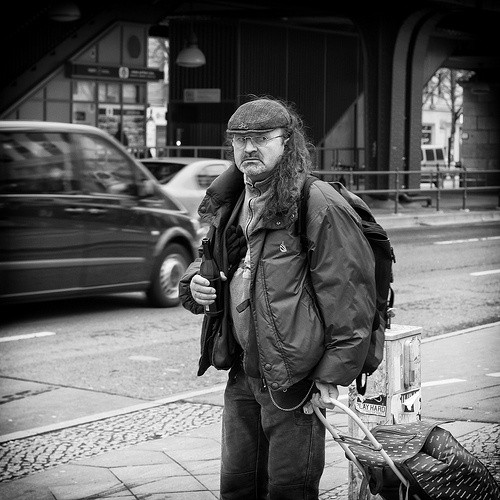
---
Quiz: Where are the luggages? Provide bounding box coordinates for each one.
[313,398,500,500]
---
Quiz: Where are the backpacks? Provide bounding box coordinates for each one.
[298,177,396,396]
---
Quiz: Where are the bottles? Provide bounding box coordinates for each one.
[200,238,224,319]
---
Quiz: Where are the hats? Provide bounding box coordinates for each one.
[227,100,290,134]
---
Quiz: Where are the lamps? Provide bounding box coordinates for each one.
[175,32,208,68]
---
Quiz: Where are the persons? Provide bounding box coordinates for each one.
[177,96,375,500]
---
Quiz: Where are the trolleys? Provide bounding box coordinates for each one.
[307,394,500,500]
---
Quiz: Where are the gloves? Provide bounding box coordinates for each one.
[226,225,246,264]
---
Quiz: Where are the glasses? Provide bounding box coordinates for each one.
[233,136,286,149]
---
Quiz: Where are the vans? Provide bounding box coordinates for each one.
[420,145,446,188]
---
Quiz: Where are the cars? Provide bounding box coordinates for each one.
[126,153,253,255]
[0,118,204,307]
